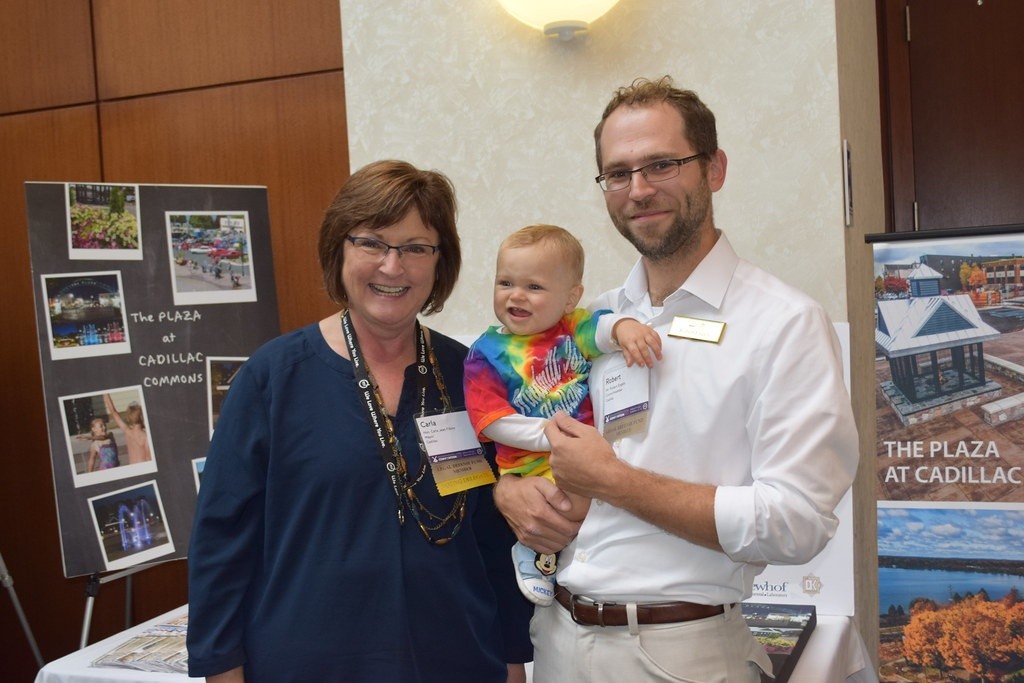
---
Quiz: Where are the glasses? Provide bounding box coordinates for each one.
[345,234,442,261]
[594,153,705,192]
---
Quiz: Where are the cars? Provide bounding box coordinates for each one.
[218,251,243,259]
[207,249,229,256]
[190,245,216,255]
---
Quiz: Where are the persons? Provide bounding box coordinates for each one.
[75,418,120,475]
[184,157,534,683]
[493,72,859,682]
[102,393,152,464]
[463,223,663,608]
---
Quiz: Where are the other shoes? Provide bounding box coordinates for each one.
[510,542,560,606]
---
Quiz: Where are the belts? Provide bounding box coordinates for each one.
[553,581,737,627]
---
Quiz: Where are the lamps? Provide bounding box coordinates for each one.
[500,0,620,42]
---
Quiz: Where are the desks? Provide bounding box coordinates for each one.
[33,597,881,682]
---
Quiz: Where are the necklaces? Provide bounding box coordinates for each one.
[363,326,469,545]
